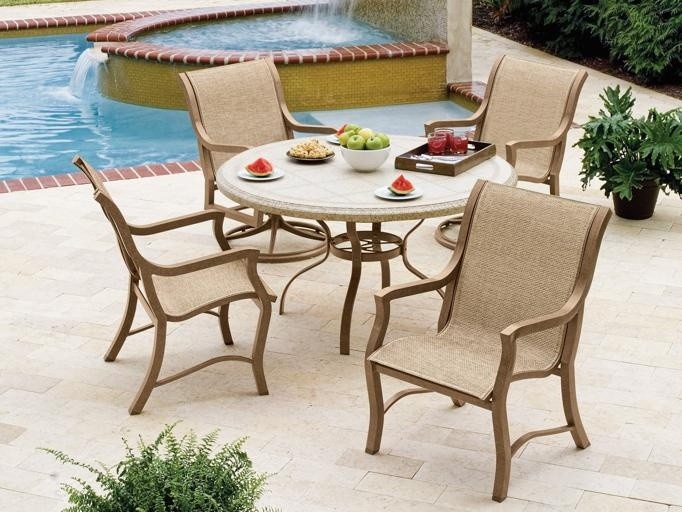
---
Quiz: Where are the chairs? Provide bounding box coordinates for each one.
[178,55,338,263]
[423,54,588,250]
[71,155,277,415]
[364,179,619,502]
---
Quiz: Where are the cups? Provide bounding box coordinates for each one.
[427,127,468,155]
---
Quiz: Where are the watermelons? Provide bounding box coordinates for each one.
[336,124,347,137]
[245,157,273,177]
[387,175,415,195]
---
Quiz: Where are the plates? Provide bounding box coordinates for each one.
[325,134,342,144]
[376,187,424,201]
[286,150,336,162]
[237,167,286,181]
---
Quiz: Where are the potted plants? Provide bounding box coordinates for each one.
[572,82,682,220]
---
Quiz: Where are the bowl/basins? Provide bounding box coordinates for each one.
[340,144,391,173]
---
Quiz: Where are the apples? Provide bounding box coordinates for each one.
[339,125,389,150]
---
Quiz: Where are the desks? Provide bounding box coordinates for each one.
[217,131,519,357]
